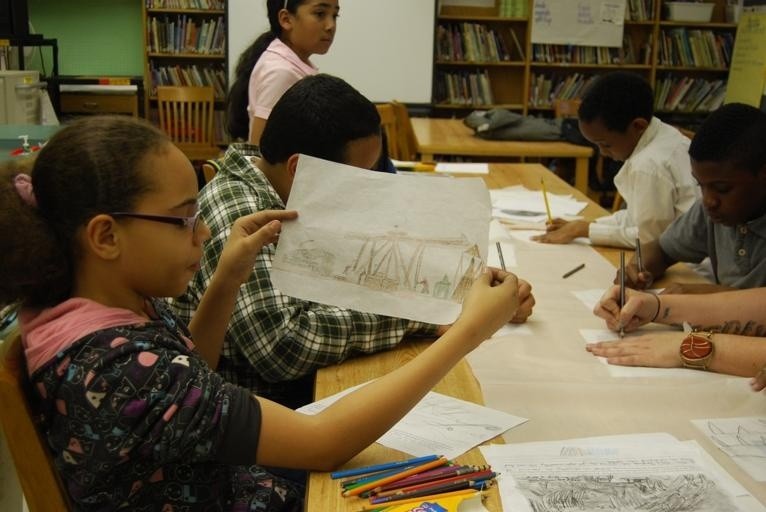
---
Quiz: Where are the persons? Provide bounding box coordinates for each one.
[614,102,766,295]
[749,365,766,391]
[530,70,717,283]
[152,73,536,395]
[0,115,519,512]
[586,284,766,378]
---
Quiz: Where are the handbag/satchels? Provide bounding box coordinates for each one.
[463,107,565,142]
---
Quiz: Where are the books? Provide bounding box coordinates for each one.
[443,67,494,106]
[657,28,734,68]
[653,78,727,111]
[147,14,225,54]
[624,0,655,21]
[148,59,226,97]
[147,0,226,10]
[435,21,525,63]
[498,0,528,17]
[529,72,584,106]
[533,33,652,66]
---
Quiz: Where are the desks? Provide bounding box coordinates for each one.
[60,88,139,119]
[303,163,766,512]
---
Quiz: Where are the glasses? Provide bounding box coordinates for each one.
[110,202,203,246]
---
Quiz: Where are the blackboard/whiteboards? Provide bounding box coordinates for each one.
[224,0,438,114]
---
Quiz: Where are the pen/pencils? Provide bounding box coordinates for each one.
[541,178,552,225]
[619,252,625,340]
[496,242,505,271]
[500,219,546,232]
[563,264,584,278]
[636,239,642,272]
[331,455,501,512]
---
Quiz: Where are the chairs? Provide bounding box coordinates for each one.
[611,127,698,215]
[201,162,218,183]
[370,100,594,195]
[157,86,221,161]
[1,327,88,512]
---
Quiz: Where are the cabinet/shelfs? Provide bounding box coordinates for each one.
[141,0,229,146]
[433,1,740,196]
